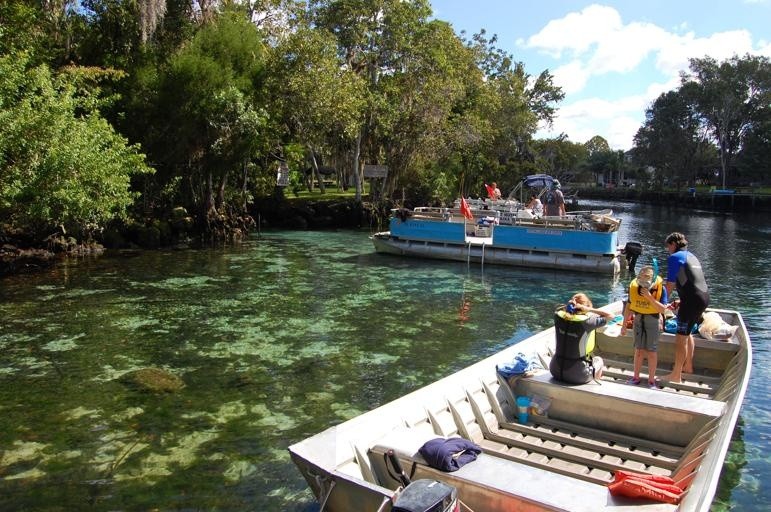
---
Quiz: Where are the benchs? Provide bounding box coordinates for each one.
[368,425,679,512]
[516,369,729,446]
[596,321,740,370]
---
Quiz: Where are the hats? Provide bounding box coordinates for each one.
[550,179,561,189]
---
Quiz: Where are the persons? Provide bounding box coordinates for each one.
[654,231,710,383]
[549,293,614,385]
[488,182,501,207]
[541,178,566,227]
[481,197,488,210]
[621,266,668,388]
[526,194,542,215]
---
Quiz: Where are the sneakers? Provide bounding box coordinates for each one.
[629,376,658,389]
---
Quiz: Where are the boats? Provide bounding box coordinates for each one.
[287,296,753,512]
[369,173,621,275]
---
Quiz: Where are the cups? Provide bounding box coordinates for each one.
[516,396,530,425]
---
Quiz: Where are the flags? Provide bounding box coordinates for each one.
[461,197,474,221]
[485,185,494,199]
